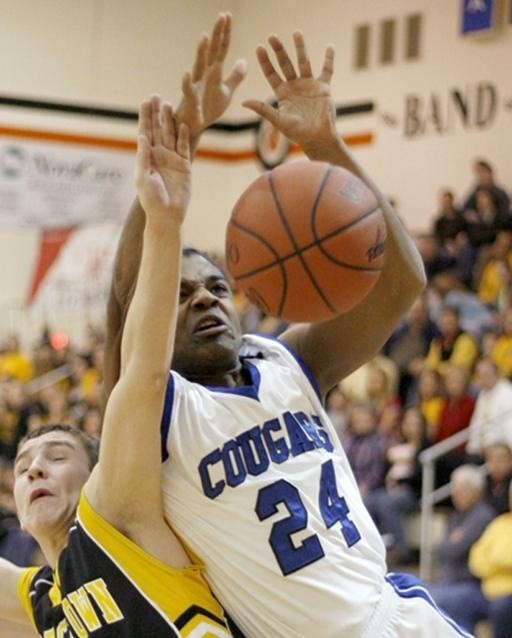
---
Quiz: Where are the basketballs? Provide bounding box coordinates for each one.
[224,160,387,323]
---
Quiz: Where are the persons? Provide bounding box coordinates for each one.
[105,15,478,638]
[3,94,243,638]
[1,322,106,568]
[320,157,512,638]
